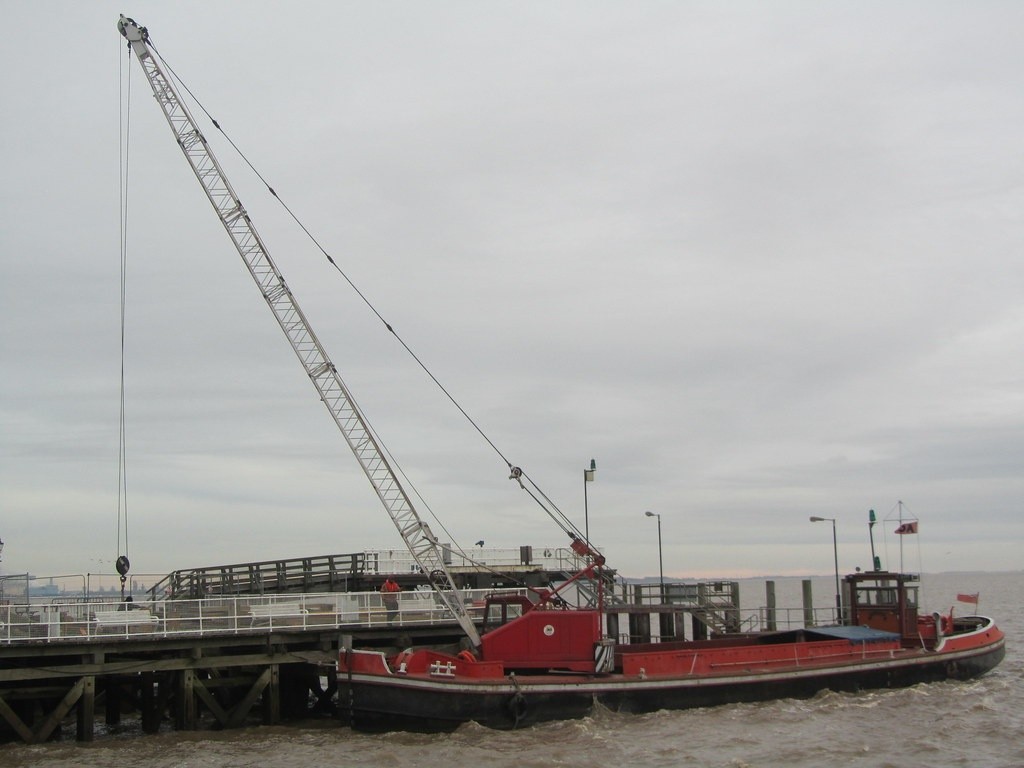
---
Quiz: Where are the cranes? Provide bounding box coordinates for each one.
[116,10,613,674]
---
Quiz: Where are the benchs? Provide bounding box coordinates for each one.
[397,598,445,621]
[248,603,308,628]
[93,610,159,633]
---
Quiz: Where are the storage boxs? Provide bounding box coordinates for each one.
[917,623,935,636]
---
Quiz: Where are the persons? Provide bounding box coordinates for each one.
[380,575,402,626]
[117,596,145,611]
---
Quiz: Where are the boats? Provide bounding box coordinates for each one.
[334,500,1005,735]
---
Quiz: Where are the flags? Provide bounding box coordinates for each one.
[894,522,917,534]
[958,594,977,603]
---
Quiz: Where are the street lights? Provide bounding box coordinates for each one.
[809,516,843,626]
[644,511,664,605]
[584,459,597,547]
